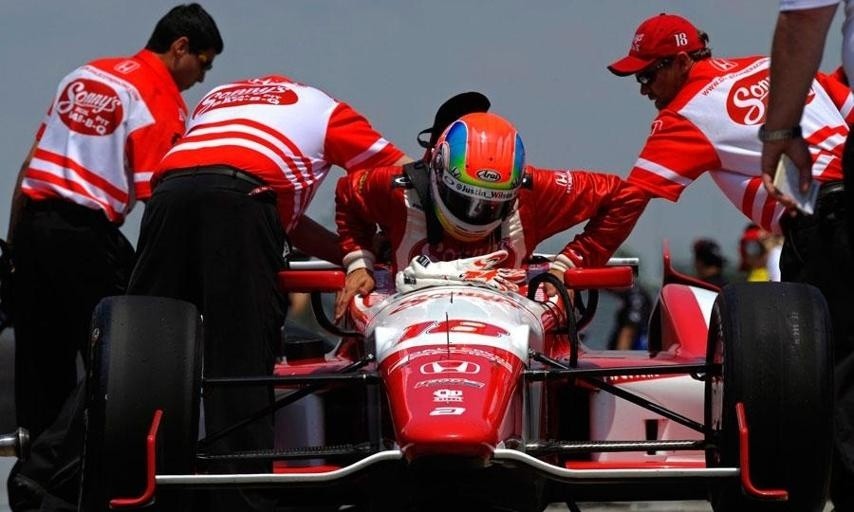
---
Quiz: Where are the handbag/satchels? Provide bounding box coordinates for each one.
[7,383,107,510]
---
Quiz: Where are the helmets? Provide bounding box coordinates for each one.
[427,113,525,242]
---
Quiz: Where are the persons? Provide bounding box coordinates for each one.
[140,70,413,472]
[332,109,625,321]
[760,0,854,229]
[609,224,783,351]
[546,17,854,293]
[10,2,226,454]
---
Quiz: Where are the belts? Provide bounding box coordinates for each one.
[163,167,260,186]
[819,181,845,197]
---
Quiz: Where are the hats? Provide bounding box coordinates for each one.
[607,13,706,77]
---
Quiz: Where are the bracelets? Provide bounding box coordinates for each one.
[758,120,802,144]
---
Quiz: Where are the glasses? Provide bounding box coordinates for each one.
[634,59,668,85]
